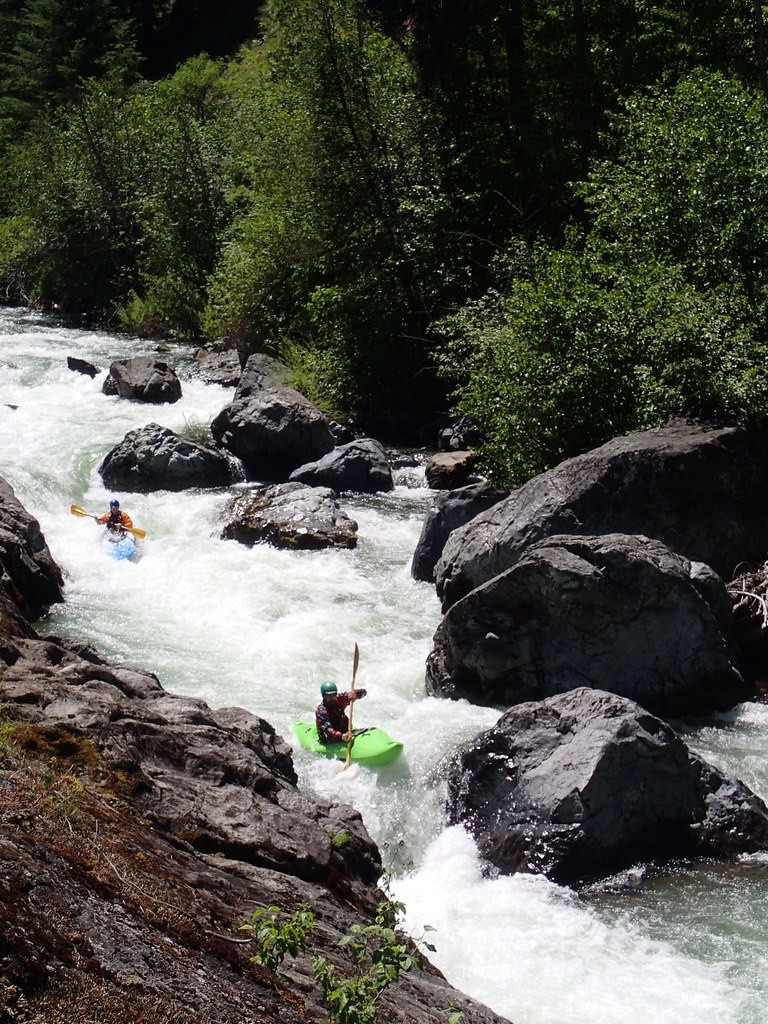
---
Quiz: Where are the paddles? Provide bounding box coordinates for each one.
[339,642,361,772]
[70,504,146,540]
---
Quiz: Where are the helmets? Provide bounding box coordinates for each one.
[111,500,120,508]
[321,681,337,694]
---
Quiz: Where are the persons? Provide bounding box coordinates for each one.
[315,681,366,745]
[95,499,133,533]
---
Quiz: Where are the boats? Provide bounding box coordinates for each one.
[296,718,403,765]
[88,514,138,561]
